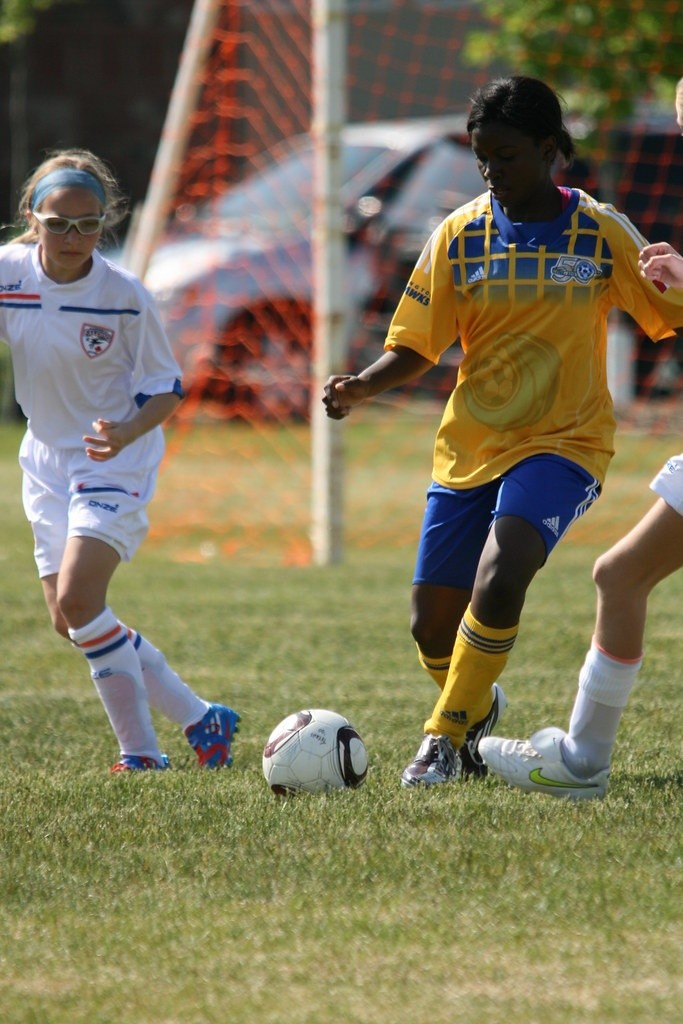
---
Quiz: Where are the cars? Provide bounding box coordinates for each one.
[85,113,682,424]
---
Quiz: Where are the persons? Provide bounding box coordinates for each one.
[0,148,242,776]
[475,74,683,799]
[321,74,683,788]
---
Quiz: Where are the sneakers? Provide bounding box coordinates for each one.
[184,703,242,771]
[400,732,457,789]
[109,753,170,778]
[477,725,612,802]
[457,681,508,783]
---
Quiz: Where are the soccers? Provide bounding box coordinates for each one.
[260,708,370,798]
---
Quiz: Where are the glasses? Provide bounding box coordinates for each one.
[30,211,107,235]
